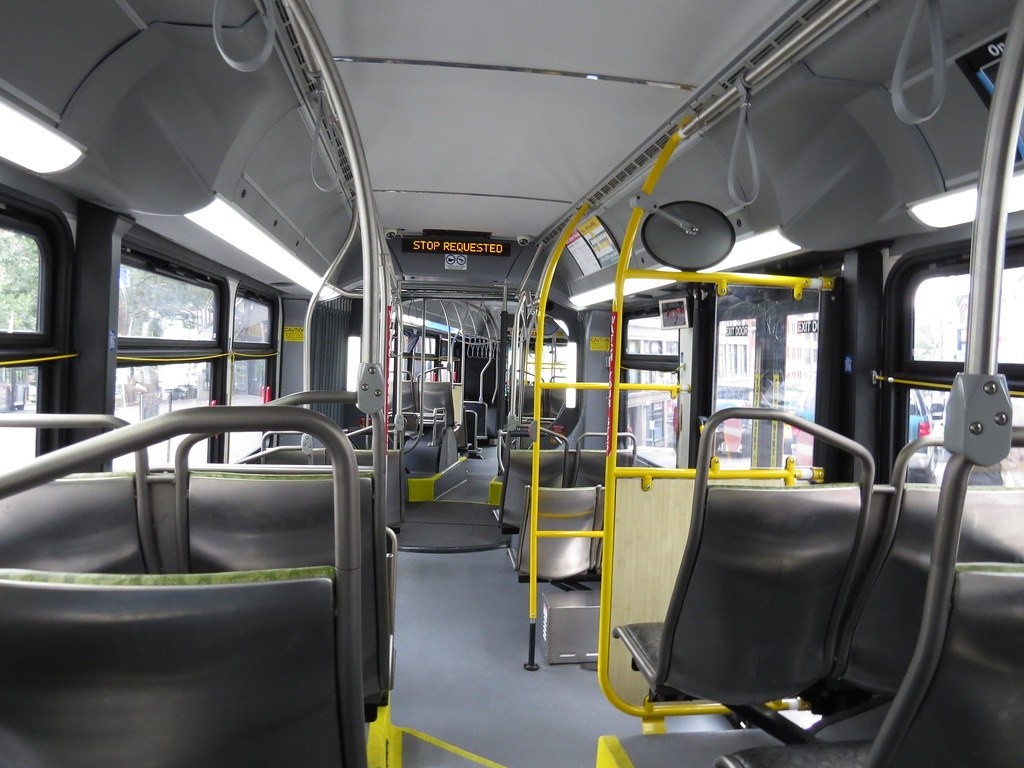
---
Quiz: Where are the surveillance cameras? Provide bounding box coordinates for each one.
[517,235,530,246]
[385,228,397,239]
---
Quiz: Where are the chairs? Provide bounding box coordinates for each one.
[386,366,461,432]
[612,407,1024,768]
[0,389,397,768]
[489,369,638,584]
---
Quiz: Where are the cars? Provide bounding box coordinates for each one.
[792,381,945,482]
[669,377,805,457]
